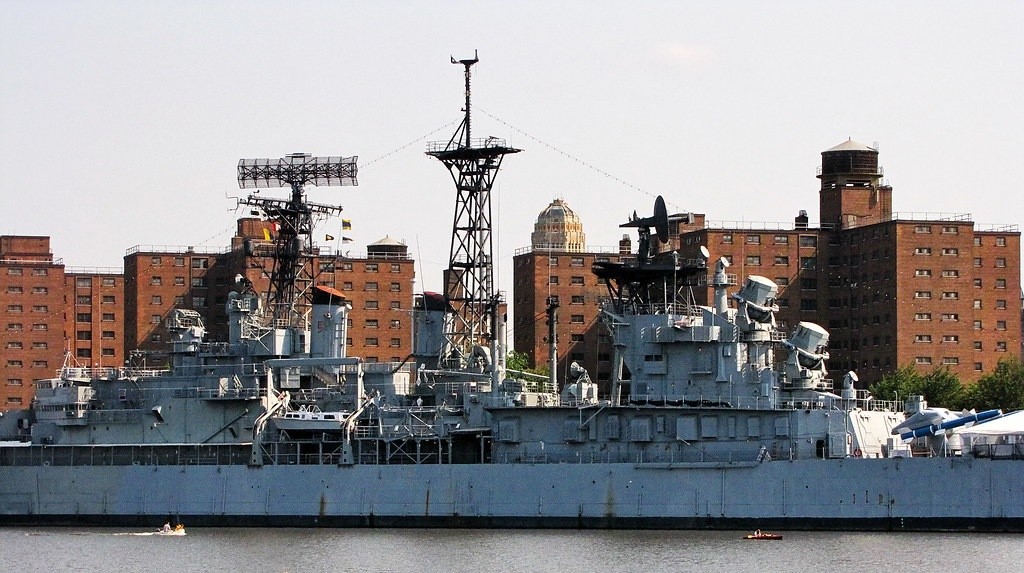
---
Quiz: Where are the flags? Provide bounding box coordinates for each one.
[342,218,351,230]
[325,234,334,241]
[263,222,281,242]
[342,236,357,246]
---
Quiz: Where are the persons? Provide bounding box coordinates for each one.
[17,416,29,434]
[155,522,184,532]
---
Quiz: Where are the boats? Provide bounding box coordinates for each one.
[271,409,352,429]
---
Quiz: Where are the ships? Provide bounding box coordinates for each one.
[0,50,1024,528]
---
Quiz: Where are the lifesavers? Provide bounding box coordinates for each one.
[854,448,862,457]
